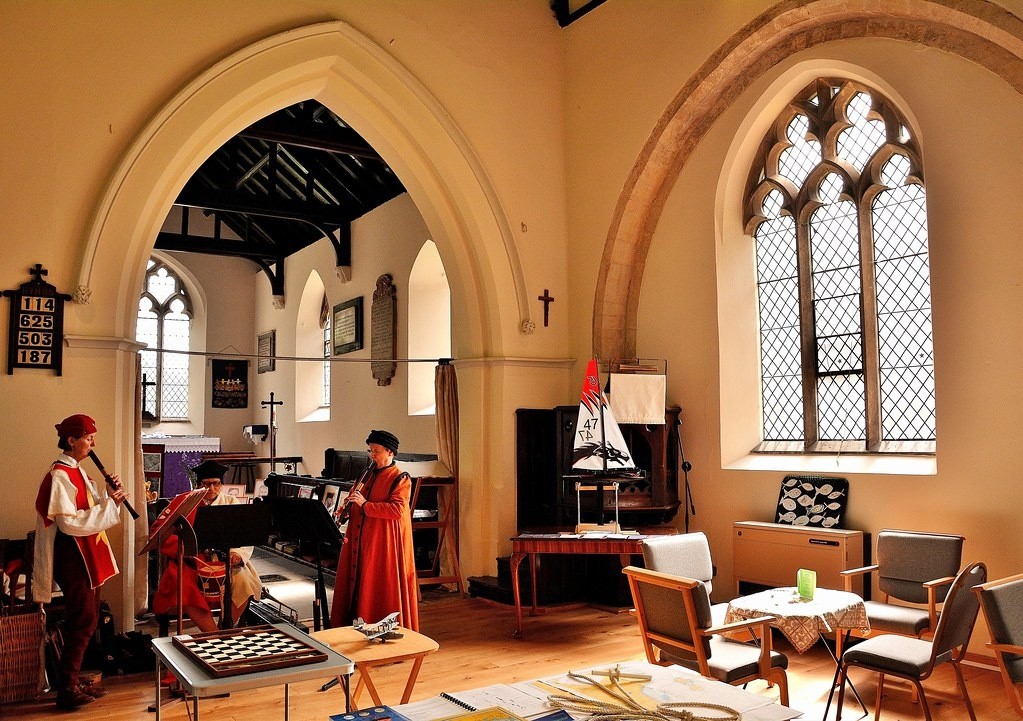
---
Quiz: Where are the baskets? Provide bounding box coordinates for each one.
[0,603,44,704]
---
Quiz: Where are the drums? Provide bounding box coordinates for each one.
[197,566,227,602]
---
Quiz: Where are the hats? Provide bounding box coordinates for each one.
[366,430,399,456]
[55,414,97,438]
[191,460,229,485]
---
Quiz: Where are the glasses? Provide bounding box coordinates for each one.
[201,481,222,488]
[367,449,381,454]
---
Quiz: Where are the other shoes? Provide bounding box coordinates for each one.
[78,683,108,696]
[55,687,95,708]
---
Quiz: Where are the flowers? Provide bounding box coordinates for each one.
[176,452,202,486]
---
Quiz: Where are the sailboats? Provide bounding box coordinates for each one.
[558,353,646,486]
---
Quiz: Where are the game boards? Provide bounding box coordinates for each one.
[171,624,329,680]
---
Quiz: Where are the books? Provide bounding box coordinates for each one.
[329,704,413,721]
[390,683,559,721]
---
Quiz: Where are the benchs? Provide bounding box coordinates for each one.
[266,448,438,565]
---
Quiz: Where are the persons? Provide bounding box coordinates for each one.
[330,430,422,634]
[154,459,263,633]
[31,414,132,705]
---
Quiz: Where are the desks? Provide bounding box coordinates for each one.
[307,623,439,712]
[510,525,680,639]
[329,659,804,721]
[151,622,354,721]
[723,586,872,721]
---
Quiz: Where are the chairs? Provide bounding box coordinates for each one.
[622,565,791,721]
[836,561,987,721]
[971,574,1023,718]
[836,528,966,702]
[636,531,775,688]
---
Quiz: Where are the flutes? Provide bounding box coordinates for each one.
[335,460,376,525]
[88,451,142,519]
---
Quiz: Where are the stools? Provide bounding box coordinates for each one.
[231,463,256,494]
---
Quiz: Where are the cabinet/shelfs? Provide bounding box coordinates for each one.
[515,406,682,607]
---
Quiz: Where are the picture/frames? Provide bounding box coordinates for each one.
[333,296,364,356]
